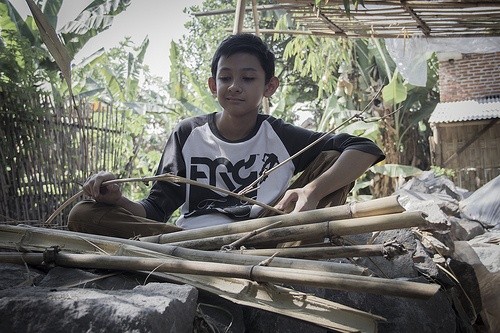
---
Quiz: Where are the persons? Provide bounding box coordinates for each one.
[68,31,387,333]
